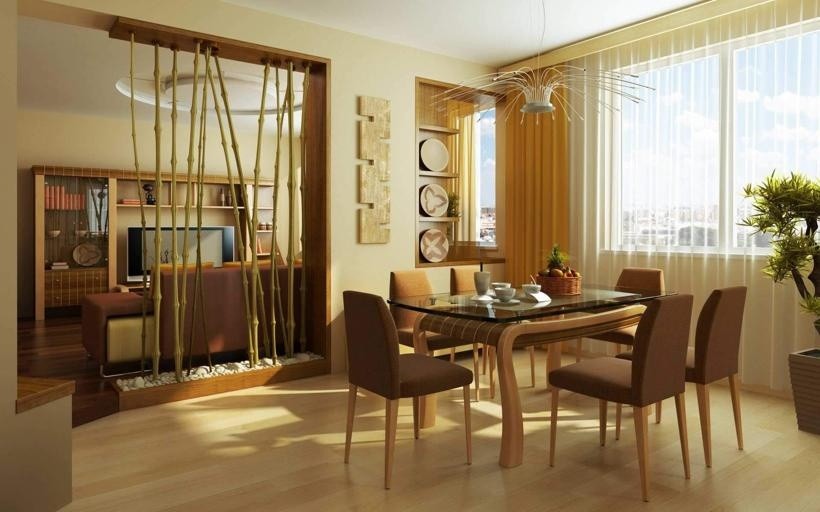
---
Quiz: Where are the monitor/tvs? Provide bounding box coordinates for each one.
[126,225,234,283]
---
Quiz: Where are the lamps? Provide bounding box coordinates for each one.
[430,1,658,124]
[166,70,283,111]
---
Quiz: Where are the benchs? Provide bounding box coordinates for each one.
[15,379,77,512]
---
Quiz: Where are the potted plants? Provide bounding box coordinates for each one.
[737,170,820,438]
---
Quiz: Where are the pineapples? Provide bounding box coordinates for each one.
[546,245,568,272]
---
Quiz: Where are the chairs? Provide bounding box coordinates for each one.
[575,268,665,361]
[390,271,455,364]
[615,285,747,467]
[449,267,494,374]
[343,291,474,490]
[544,293,694,502]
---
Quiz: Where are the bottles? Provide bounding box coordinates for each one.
[220,188,225,206]
[226,188,233,206]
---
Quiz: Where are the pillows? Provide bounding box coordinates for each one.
[148,262,216,297]
[223,260,272,271]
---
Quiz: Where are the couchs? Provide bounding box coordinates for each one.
[81,264,306,380]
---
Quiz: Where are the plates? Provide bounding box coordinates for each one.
[420,229,450,262]
[422,138,449,171]
[72,244,102,266]
[472,299,491,305]
[420,183,449,217]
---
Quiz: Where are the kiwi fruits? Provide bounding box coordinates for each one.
[538,269,550,276]
[550,269,564,278]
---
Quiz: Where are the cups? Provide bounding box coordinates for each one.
[474,272,489,295]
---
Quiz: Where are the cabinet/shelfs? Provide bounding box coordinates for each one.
[32,166,110,320]
[117,170,288,294]
[420,124,463,262]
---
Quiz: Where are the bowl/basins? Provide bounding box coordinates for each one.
[492,283,511,294]
[522,285,542,301]
[495,287,516,302]
[48,231,60,237]
